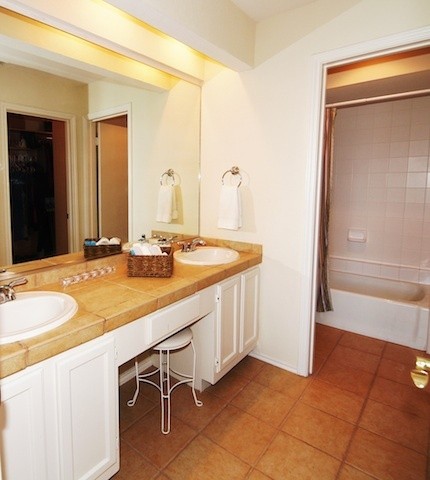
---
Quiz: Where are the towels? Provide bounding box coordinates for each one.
[215,185,242,231]
[156,186,179,223]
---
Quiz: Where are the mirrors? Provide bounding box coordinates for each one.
[0,7,203,280]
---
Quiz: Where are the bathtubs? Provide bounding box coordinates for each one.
[314,269,430,355]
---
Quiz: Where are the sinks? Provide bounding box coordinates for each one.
[0,271,22,280]
[0,290,77,345]
[173,246,240,265]
[122,238,171,251]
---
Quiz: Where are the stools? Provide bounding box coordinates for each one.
[127,327,203,434]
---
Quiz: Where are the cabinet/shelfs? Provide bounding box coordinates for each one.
[0,332,121,480]
[214,264,261,396]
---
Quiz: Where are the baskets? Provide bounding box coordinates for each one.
[127,247,173,278]
[83,237,121,259]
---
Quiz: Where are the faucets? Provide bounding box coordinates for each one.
[157,236,177,246]
[177,238,206,252]
[138,234,150,244]
[0,278,28,304]
